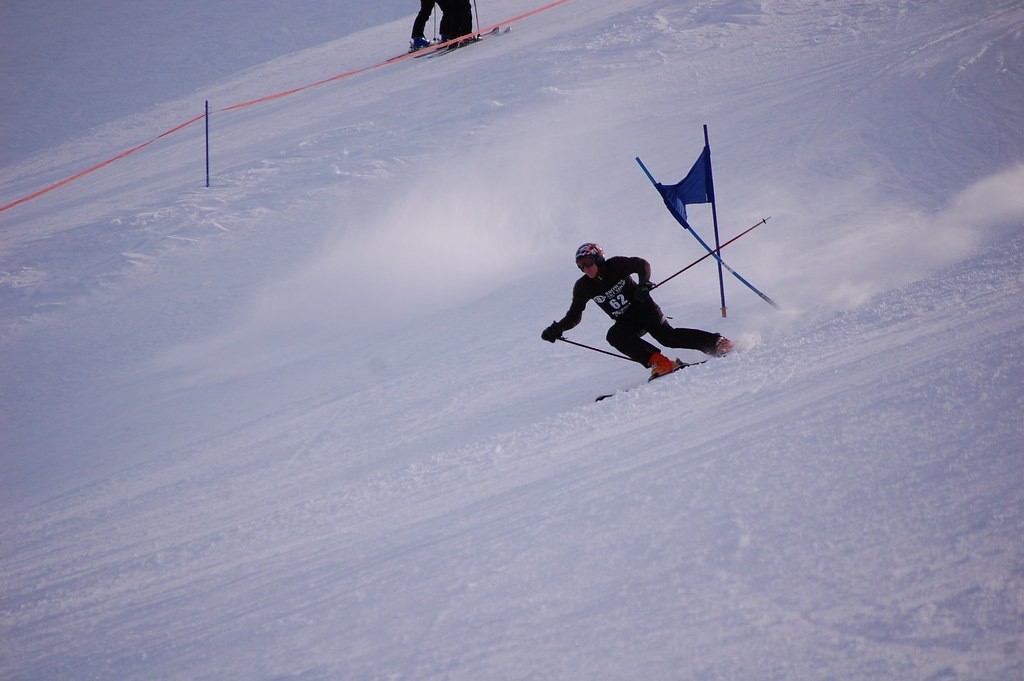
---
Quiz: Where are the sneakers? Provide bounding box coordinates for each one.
[713,336,736,358]
[649,352,676,376]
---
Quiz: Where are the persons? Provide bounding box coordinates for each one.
[542,243,732,382]
[408,0,483,50]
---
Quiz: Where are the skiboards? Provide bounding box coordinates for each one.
[594,348,734,402]
[387,24,512,59]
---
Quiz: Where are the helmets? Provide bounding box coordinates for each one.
[575,243,604,273]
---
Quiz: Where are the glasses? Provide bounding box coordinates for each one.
[578,257,596,271]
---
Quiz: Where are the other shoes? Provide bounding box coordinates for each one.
[411,37,434,48]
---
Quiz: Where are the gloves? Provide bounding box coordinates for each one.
[541,321,563,343]
[635,281,652,303]
